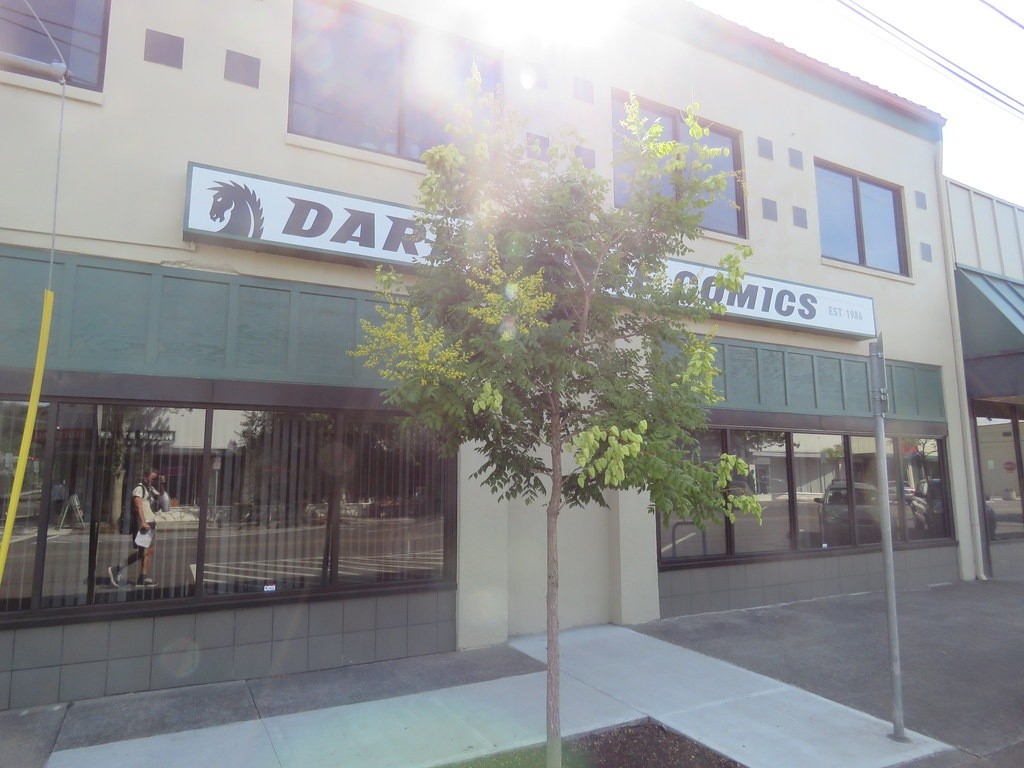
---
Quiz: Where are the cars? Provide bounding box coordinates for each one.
[0,488,43,522]
[889,478,914,504]
[377,491,425,518]
[730,480,752,496]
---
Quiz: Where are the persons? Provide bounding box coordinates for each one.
[109,469,165,588]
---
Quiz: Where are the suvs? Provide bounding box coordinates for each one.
[815,479,882,544]
[911,477,998,536]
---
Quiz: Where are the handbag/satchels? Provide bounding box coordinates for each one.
[133,526,155,548]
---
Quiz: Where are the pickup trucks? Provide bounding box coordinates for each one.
[303,492,379,524]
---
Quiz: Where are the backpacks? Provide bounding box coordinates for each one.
[118,483,154,535]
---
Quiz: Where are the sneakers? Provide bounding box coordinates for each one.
[136,577,158,587]
[108,566,122,587]
